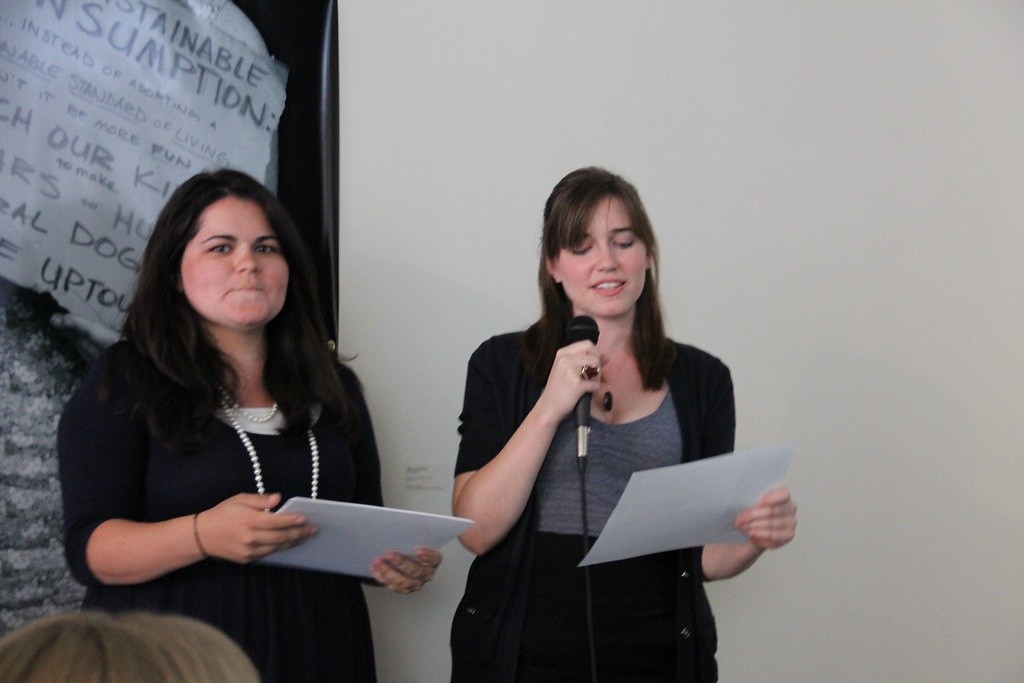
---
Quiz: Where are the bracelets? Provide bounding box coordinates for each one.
[193,513,211,559]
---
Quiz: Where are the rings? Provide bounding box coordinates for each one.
[581,365,599,380]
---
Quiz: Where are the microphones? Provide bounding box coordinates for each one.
[564,315,599,471]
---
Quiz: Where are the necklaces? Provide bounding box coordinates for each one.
[221,392,319,510]
[601,351,633,410]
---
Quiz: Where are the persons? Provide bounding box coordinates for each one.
[450,167,797,683]
[57,170,442,683]
[0,616,260,683]
